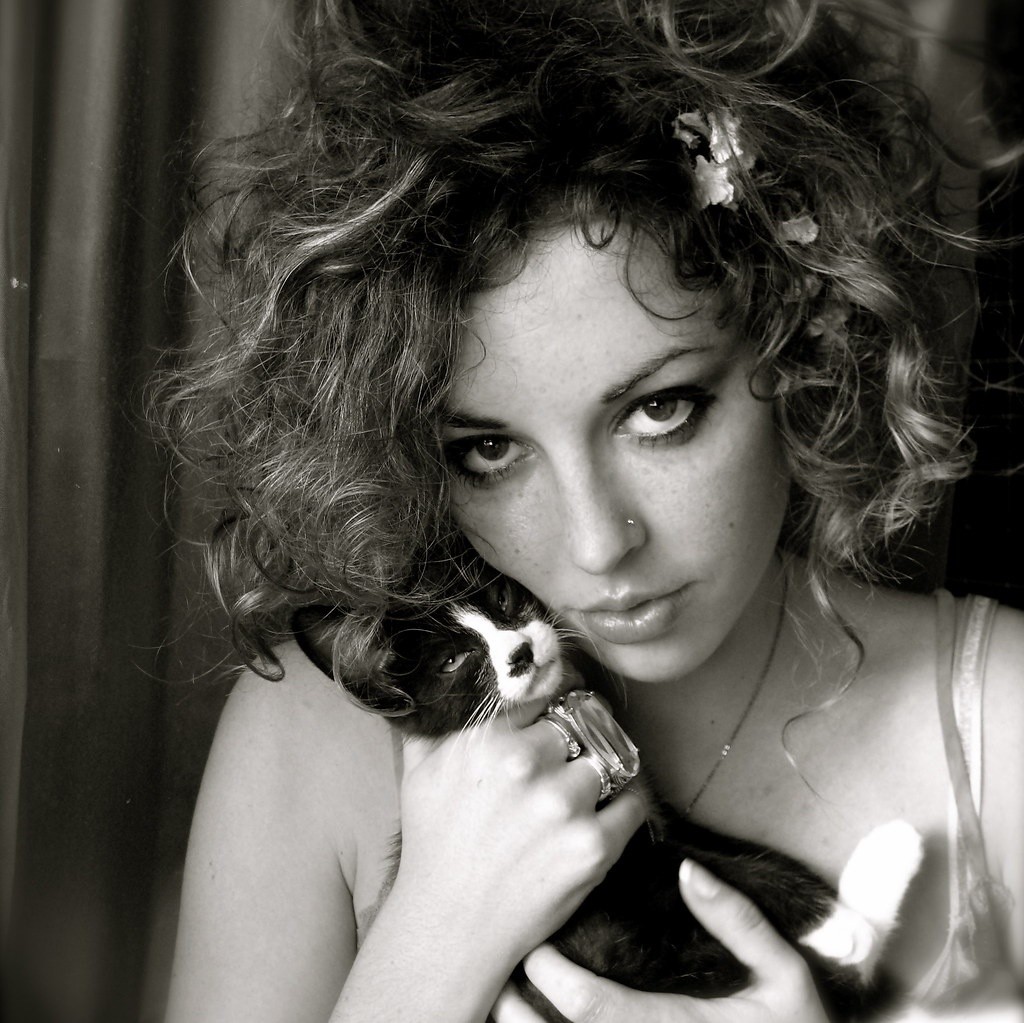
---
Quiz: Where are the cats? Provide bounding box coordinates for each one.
[290,541,902,1023]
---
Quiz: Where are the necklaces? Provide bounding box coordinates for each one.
[681,546,787,817]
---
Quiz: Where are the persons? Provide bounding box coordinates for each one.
[167,2,1024,1023]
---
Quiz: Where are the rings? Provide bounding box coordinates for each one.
[534,692,640,801]
[544,715,581,758]
